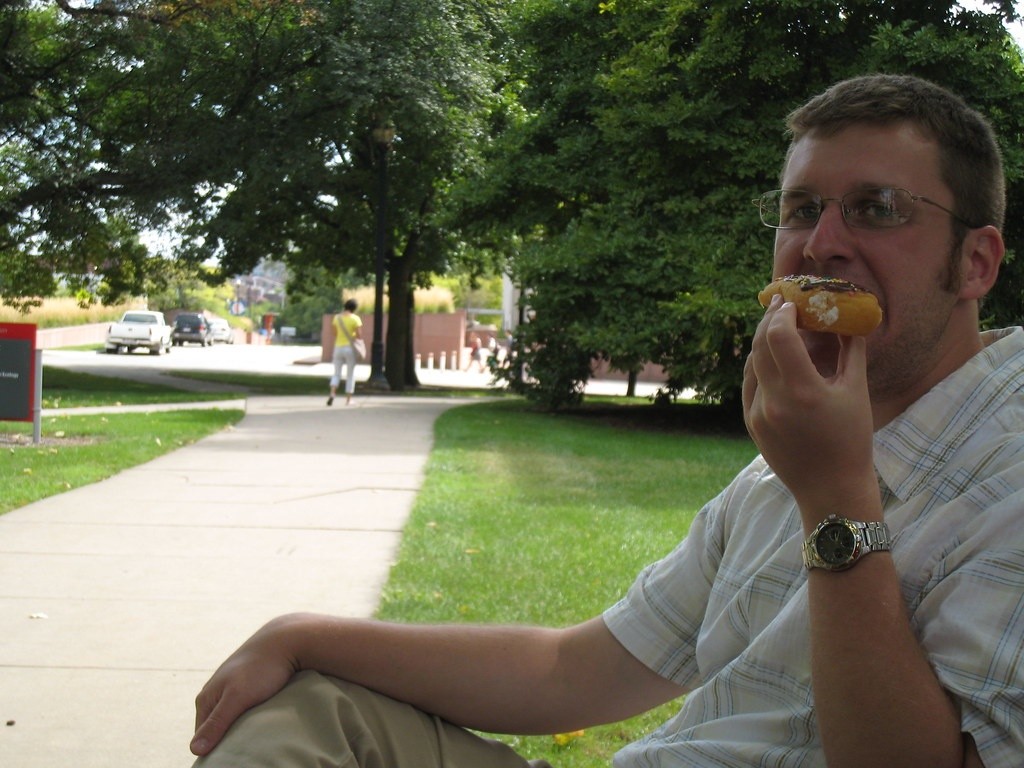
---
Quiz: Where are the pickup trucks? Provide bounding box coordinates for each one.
[104,309,173,356]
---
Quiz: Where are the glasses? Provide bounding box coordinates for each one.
[751,186,965,233]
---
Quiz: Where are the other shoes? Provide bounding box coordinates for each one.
[347,401,355,406]
[327,395,334,407]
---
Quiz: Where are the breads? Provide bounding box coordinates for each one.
[759,273,881,337]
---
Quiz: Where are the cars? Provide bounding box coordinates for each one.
[170,313,213,347]
[208,317,233,344]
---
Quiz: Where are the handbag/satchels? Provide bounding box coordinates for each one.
[351,337,367,361]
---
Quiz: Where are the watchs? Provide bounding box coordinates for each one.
[802,513,892,573]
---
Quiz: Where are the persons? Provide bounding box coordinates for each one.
[188,72,1022,766]
[326,299,363,407]
[465,331,483,373]
[480,332,499,371]
[502,331,515,368]
